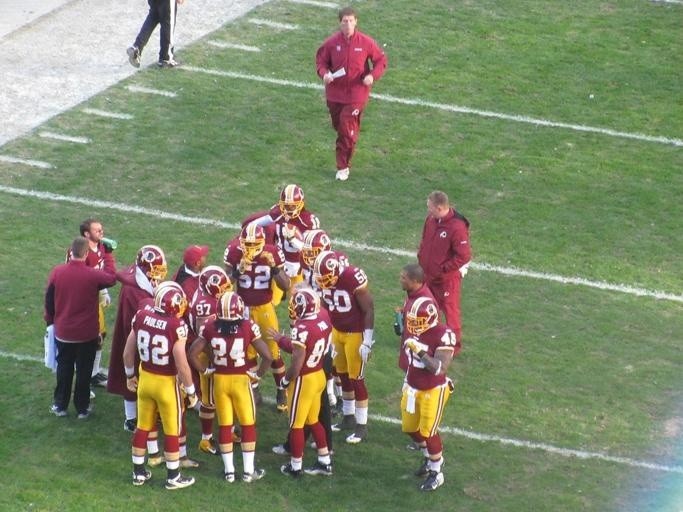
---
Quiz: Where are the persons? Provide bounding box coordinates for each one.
[417,190,472,360]
[62,220,112,400]
[42,235,118,419]
[126,0,183,70]
[393,266,439,452]
[399,295,455,492]
[107,181,372,488]
[315,7,388,181]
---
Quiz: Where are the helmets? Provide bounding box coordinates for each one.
[302,229,332,266]
[279,184,305,218]
[289,289,321,320]
[153,280,187,316]
[314,251,341,289]
[136,245,167,279]
[198,265,233,299]
[409,297,439,331]
[215,290,245,322]
[239,222,266,258]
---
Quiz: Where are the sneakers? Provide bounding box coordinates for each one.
[132,470,151,486]
[407,442,433,477]
[164,475,195,491]
[242,467,266,483]
[309,441,335,456]
[330,417,357,432]
[126,46,140,68]
[345,427,368,445]
[419,471,445,492]
[48,368,262,468]
[276,392,289,413]
[330,399,344,417]
[272,444,304,457]
[336,167,350,182]
[280,462,303,479]
[225,472,237,484]
[304,462,333,477]
[159,58,180,67]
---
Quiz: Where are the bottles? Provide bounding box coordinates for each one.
[99,237,117,250]
[394,305,403,334]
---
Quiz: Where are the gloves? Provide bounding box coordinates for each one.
[261,252,275,266]
[402,338,422,354]
[239,251,253,272]
[188,393,199,409]
[358,343,372,363]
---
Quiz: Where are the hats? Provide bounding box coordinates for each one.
[183,245,211,267]
